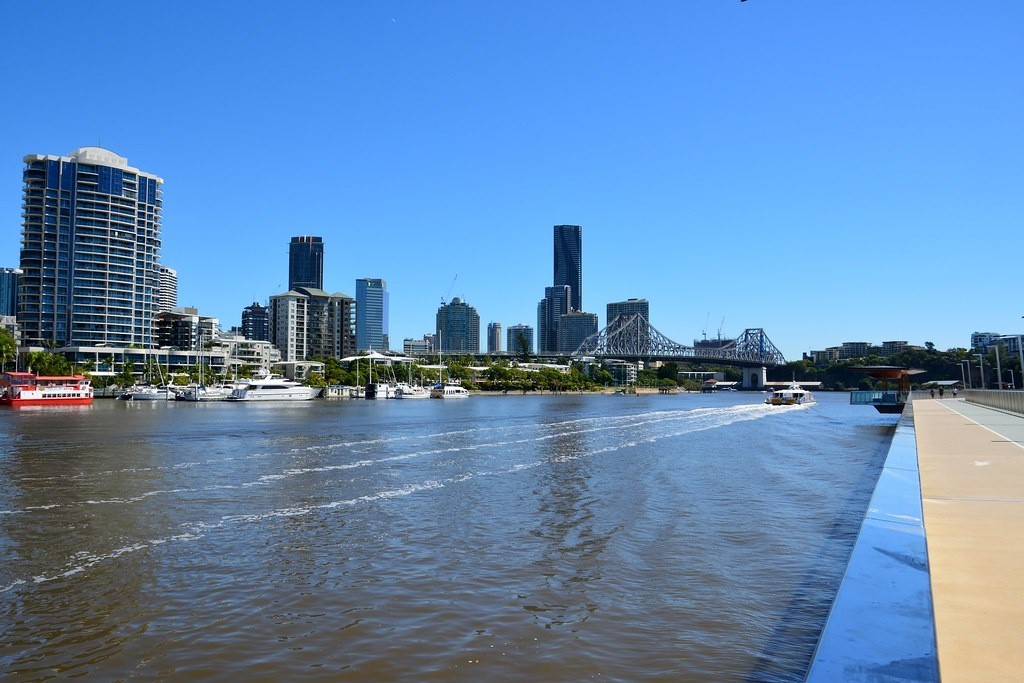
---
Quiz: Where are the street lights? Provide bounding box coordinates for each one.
[985,345,1002,389]
[972,353,984,389]
[956,363,966,389]
[960,359,972,389]
[1007,369,1014,390]
[991,334,1024,389]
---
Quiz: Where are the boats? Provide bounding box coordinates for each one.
[0,372,96,407]
[180,383,248,402]
[430,385,470,399]
[393,381,431,401]
[116,384,177,402]
[763,380,817,408]
[228,371,322,401]
